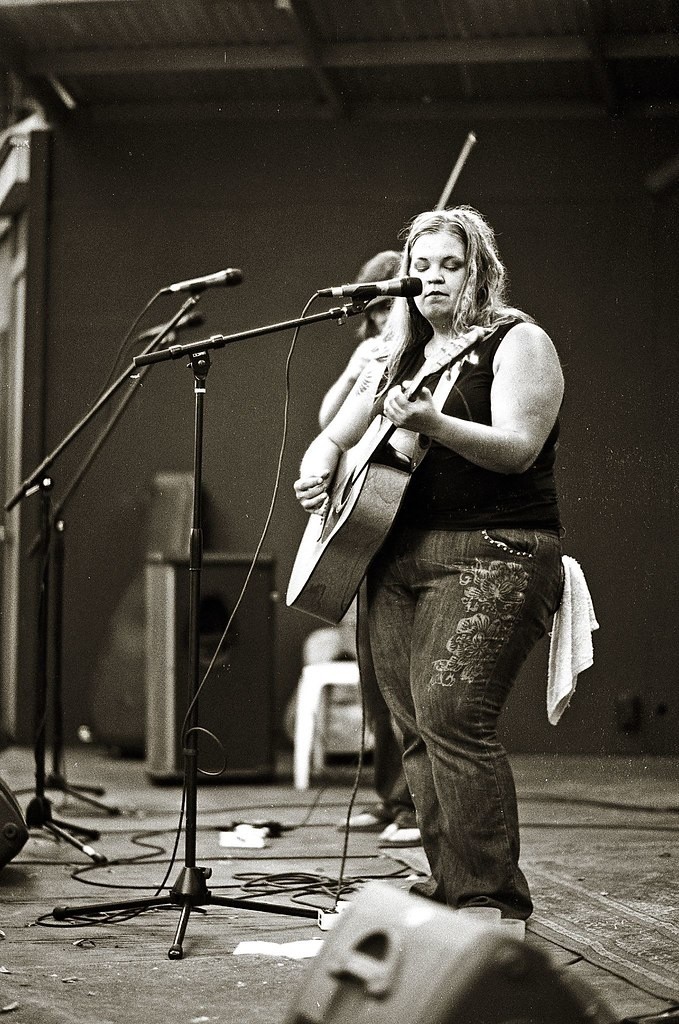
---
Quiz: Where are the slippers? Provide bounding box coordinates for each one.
[375,808,422,848]
[337,801,395,832]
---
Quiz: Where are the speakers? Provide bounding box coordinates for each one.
[284,883,601,1024]
[149,554,277,784]
[0,778,30,872]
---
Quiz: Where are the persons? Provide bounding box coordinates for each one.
[293,208,564,921]
[320,249,422,847]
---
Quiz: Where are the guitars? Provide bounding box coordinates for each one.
[285,317,483,624]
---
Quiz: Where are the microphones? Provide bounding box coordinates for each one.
[159,268,243,296]
[317,278,423,298]
[137,311,206,341]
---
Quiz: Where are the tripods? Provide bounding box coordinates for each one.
[3,291,364,960]
